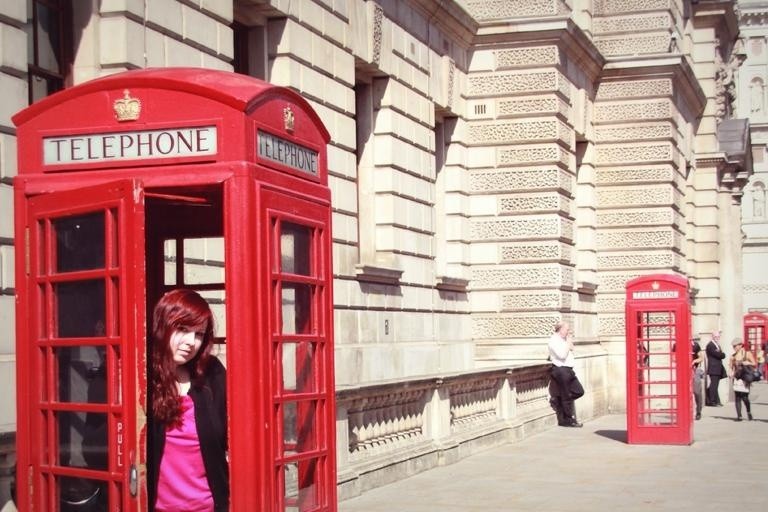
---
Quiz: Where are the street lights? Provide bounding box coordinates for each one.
[740,365,762,383]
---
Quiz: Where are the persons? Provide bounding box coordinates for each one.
[727,338,755,421]
[705,331,727,407]
[756,340,768,381]
[637,341,648,403]
[81,289,230,512]
[671,336,704,420]
[549,322,585,428]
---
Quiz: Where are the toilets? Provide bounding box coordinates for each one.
[748,342,752,351]
[637,342,649,368]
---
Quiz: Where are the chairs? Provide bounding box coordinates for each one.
[550,396,561,411]
[570,422,583,427]
